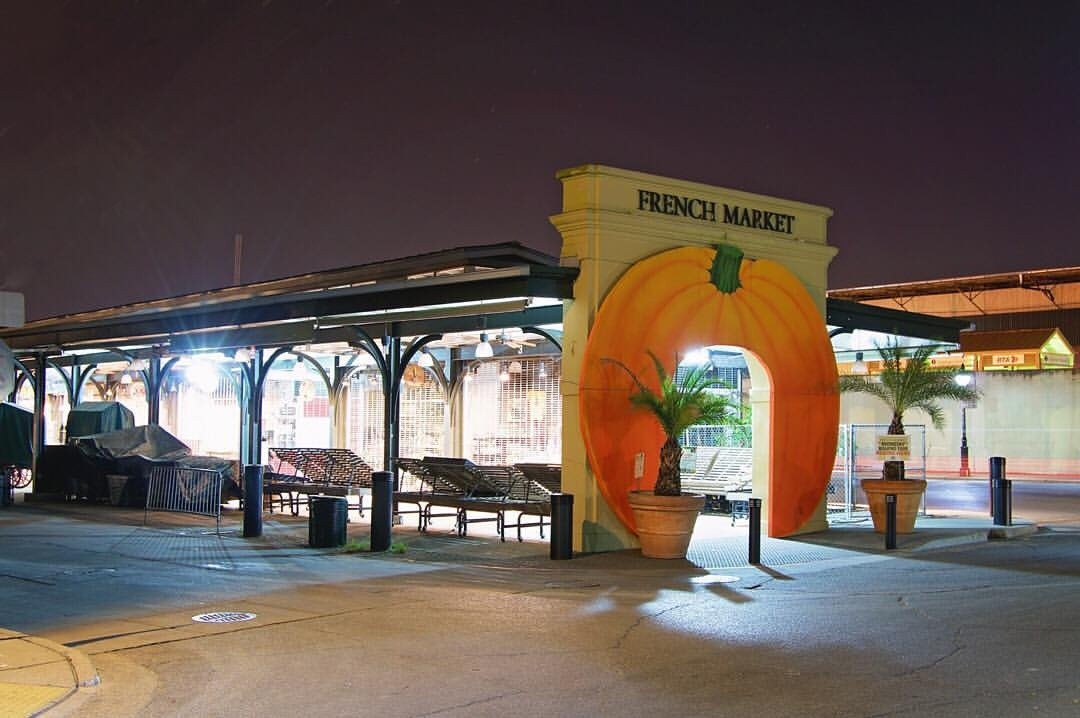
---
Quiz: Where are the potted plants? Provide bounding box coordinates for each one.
[600,351,751,559]
[836,336,983,534]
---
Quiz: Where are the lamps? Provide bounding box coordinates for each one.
[535,362,548,377]
[474,332,494,359]
[120,370,133,385]
[416,346,434,367]
[294,356,307,374]
[499,361,510,381]
[852,353,869,372]
[954,363,972,387]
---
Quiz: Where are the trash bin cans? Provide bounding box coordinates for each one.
[308,495,348,548]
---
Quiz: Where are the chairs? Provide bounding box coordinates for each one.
[679,446,753,526]
[263,448,561,544]
